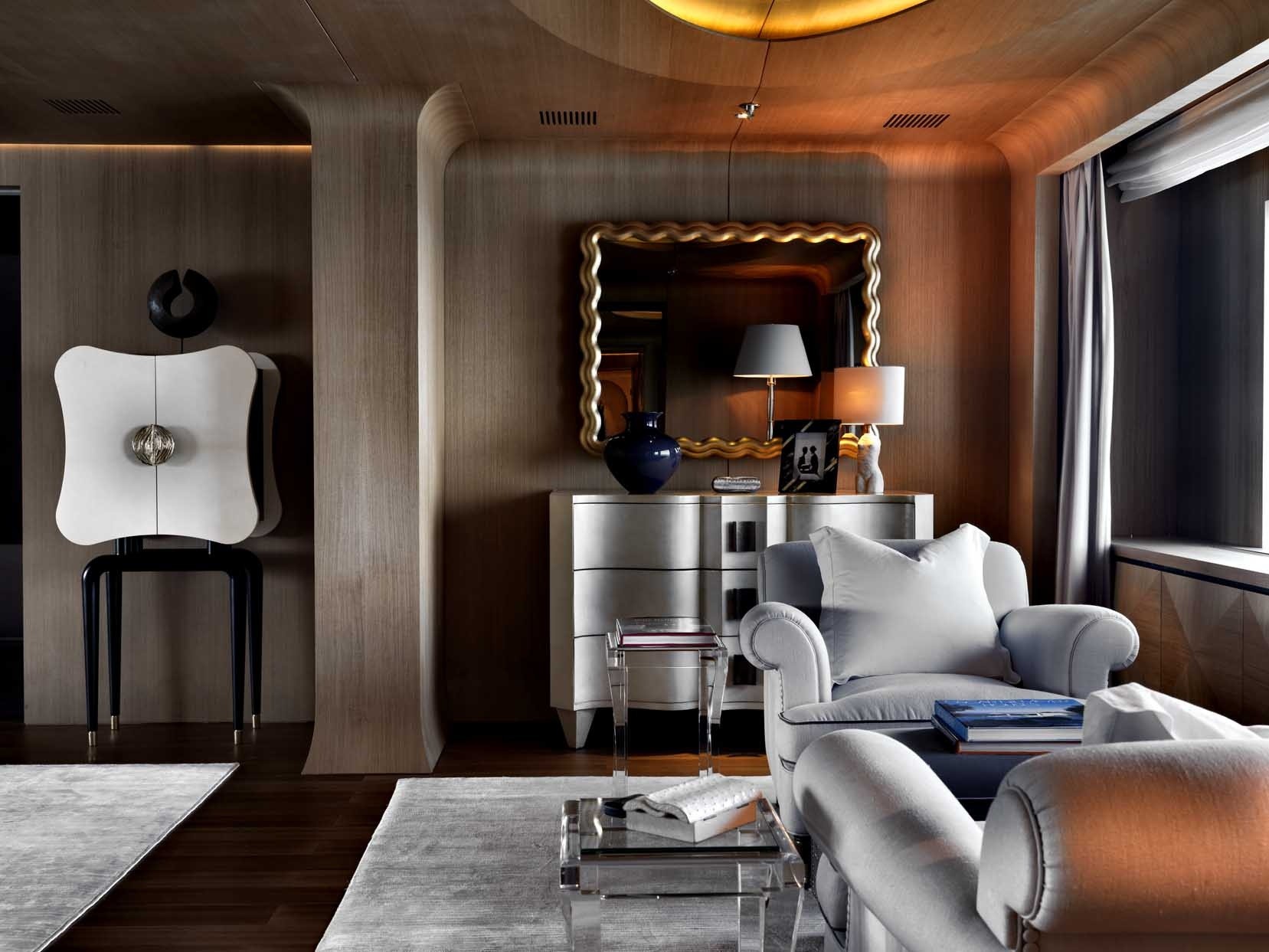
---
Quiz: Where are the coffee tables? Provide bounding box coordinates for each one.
[893,727,1082,820]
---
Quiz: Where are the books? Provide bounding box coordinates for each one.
[625,801,757,843]
[931,699,1085,754]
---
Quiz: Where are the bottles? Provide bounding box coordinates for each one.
[603,411,682,495]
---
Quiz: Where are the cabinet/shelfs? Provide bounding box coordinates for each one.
[54,346,280,743]
[549,489,934,751]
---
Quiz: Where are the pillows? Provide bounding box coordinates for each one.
[809,522,1020,689]
[1077,684,1260,743]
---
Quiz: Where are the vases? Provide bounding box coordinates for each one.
[603,411,683,495]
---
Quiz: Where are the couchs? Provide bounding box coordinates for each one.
[791,731,1268,952]
[739,540,1141,833]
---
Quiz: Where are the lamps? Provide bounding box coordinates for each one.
[834,366,906,494]
[733,324,812,442]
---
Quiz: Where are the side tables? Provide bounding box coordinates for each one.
[605,617,726,776]
[558,796,808,952]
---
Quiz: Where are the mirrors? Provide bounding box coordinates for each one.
[577,225,882,457]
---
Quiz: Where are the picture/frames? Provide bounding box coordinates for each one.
[777,420,841,499]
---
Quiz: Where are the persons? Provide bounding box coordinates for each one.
[798,445,818,473]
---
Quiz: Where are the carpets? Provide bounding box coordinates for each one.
[314,776,838,951]
[2,761,242,952]
